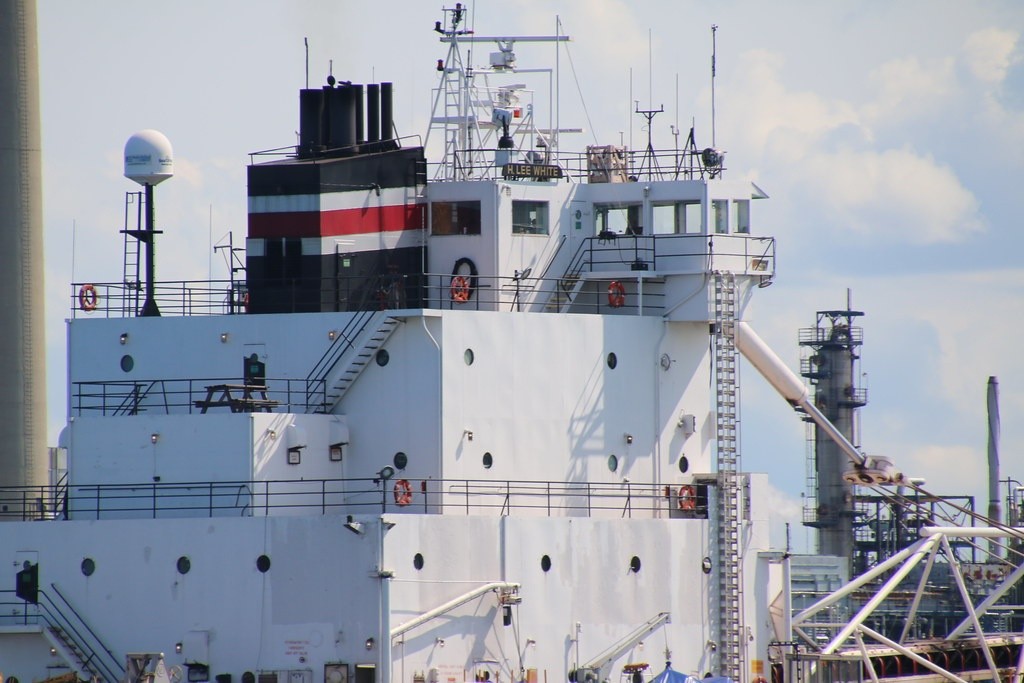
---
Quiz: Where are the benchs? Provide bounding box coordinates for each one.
[194,400,230,413]
[230,398,279,413]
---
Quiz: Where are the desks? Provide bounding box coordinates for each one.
[200,384,273,413]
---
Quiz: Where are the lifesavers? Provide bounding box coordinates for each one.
[607,280,627,306]
[393,480,413,505]
[79,284,98,310]
[679,485,697,510]
[450,276,471,303]
